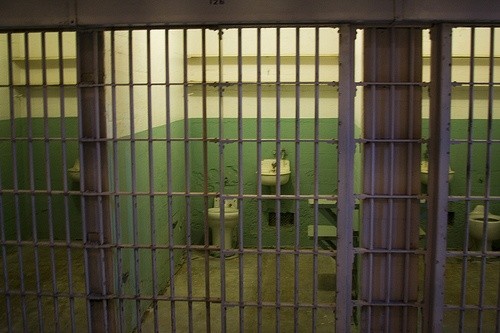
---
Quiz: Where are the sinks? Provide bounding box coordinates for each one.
[421,160,456,187]
[254,158,292,187]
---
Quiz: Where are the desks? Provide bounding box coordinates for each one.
[309,198,360,230]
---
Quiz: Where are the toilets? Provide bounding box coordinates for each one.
[206,196,240,257]
[467,204,500,258]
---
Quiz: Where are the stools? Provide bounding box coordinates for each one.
[307,224,337,259]
